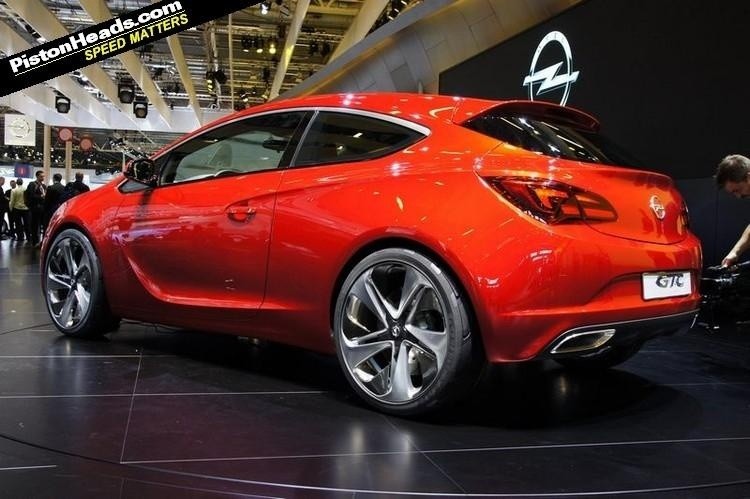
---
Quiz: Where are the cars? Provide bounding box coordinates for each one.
[35,89,709,418]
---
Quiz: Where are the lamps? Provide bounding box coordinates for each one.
[55,95,71,113]
[118,76,148,119]
[206,69,227,85]
[239,33,278,54]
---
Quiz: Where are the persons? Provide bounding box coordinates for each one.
[0,171,90,249]
[713,154,750,269]
